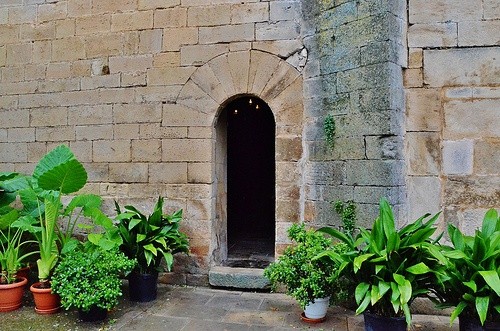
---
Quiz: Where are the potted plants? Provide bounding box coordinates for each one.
[262,222,351,324]
[0,172,45,312]
[49,247,139,322]
[9,144,113,313]
[432,208,500,331]
[312,197,449,331]
[113,195,192,303]
